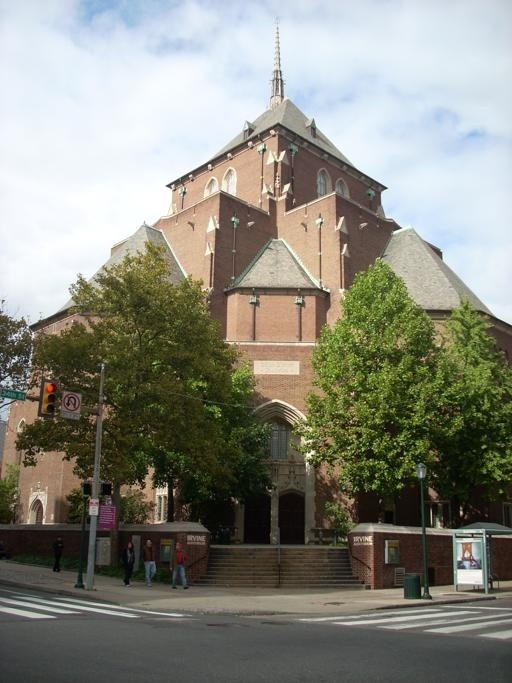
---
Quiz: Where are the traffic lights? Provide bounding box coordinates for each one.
[39,380,57,415]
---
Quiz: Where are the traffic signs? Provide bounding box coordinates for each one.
[59,408,80,420]
[0,387,26,401]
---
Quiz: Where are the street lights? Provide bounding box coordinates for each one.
[416,460,431,600]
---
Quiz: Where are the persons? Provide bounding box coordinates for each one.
[52,533,62,573]
[142,539,157,587]
[122,540,135,586]
[169,540,189,590]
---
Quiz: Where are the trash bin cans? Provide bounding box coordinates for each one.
[403,576,421,599]
